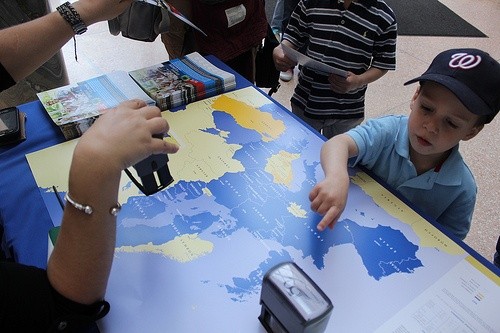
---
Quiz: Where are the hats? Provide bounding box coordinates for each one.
[403,48,500,125]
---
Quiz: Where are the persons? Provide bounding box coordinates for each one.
[309,48,500,240]
[0,98,180,333]
[0,0,132,109]
[157,0,300,97]
[273,0,397,139]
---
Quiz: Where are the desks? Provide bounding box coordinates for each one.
[0,55,500,333]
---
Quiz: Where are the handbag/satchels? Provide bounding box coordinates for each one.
[108,0,170,42]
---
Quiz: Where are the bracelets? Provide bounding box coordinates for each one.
[65,192,122,216]
[56,1,88,62]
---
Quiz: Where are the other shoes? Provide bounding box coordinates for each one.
[280,69,293,81]
